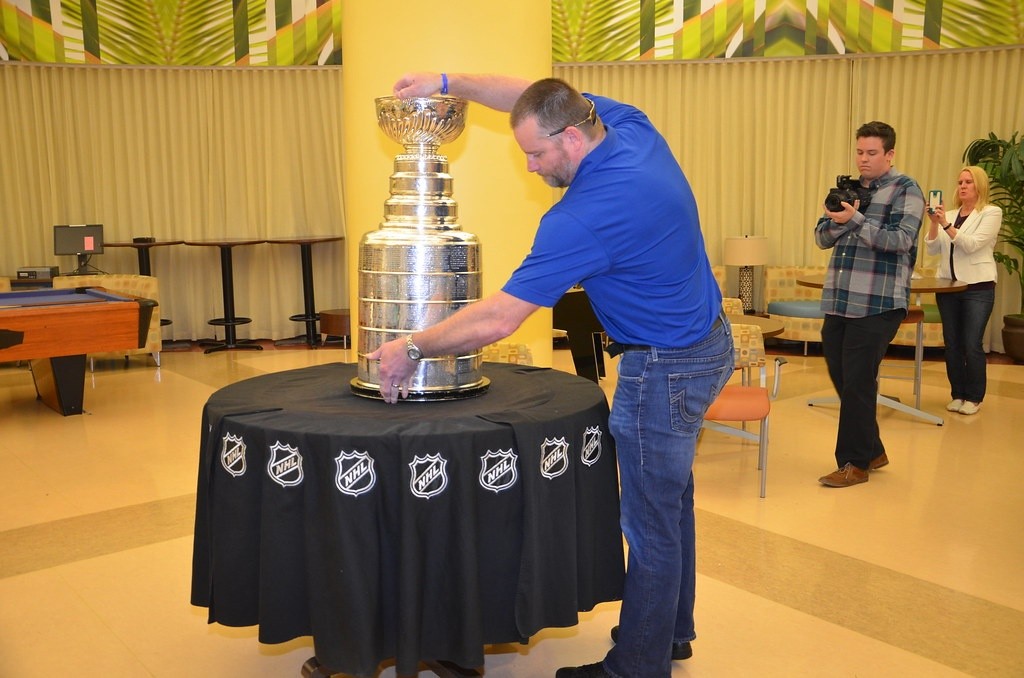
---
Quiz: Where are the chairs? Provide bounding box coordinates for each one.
[695,324,788,500]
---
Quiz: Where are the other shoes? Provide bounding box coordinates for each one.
[947,396,963,412]
[868,450,890,469]
[959,398,980,415]
[819,461,869,487]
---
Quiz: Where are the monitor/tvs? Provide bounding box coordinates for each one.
[54,225,103,276]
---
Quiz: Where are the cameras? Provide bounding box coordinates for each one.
[825,178,871,212]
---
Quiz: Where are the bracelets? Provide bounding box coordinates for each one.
[441,74,448,95]
[944,223,952,230]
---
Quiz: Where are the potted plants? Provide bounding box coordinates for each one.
[964,130,1024,366]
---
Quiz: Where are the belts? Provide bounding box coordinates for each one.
[605,310,726,359]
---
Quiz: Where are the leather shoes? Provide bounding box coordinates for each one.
[611,625,692,659]
[555,660,611,678]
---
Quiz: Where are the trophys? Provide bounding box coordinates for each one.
[350,95,490,403]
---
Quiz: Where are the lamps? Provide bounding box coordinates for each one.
[723,235,769,316]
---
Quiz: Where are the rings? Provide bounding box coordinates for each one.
[392,384,399,387]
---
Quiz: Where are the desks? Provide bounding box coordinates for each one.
[761,300,943,426]
[727,314,784,433]
[0,285,160,417]
[263,234,345,351]
[190,361,626,678]
[103,238,192,354]
[796,272,969,395]
[184,239,267,354]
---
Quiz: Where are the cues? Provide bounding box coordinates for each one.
[0,297,108,309]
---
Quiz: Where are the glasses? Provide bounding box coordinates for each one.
[546,98,597,137]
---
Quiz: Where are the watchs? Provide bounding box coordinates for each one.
[408,335,424,360]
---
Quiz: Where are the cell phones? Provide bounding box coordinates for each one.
[929,190,942,214]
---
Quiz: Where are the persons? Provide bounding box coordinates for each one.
[924,166,1002,415]
[815,121,926,487]
[365,73,733,677]
